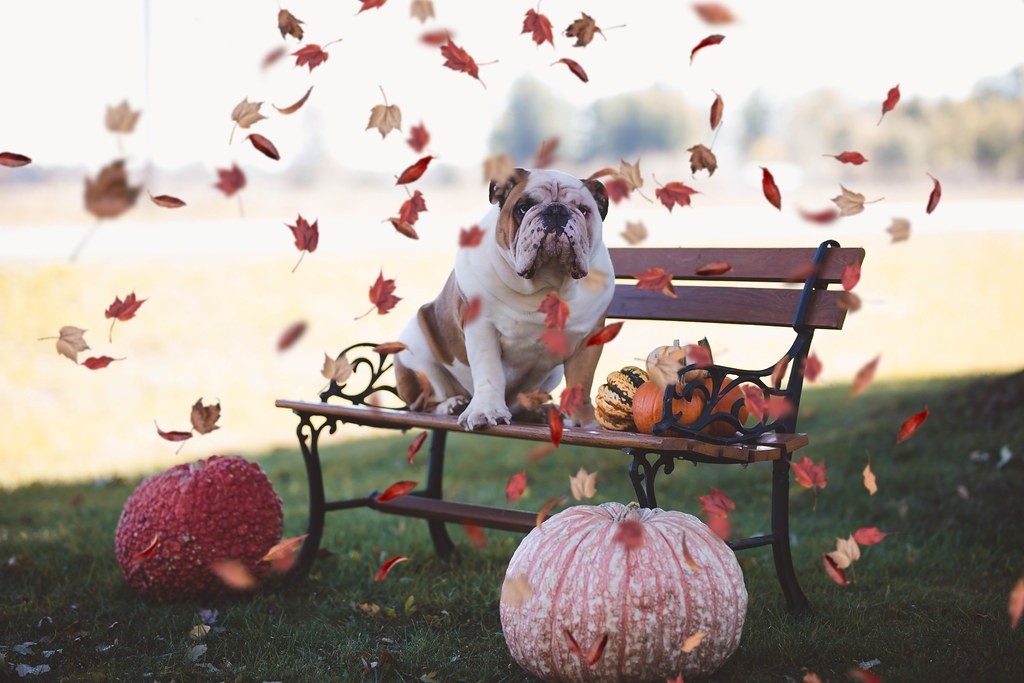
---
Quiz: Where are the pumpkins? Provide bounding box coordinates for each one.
[113,455,283,603]
[594,338,747,439]
[500,500,748,683]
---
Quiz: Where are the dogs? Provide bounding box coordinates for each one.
[389,155,613,435]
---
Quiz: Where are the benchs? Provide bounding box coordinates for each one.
[277,240,865,613]
